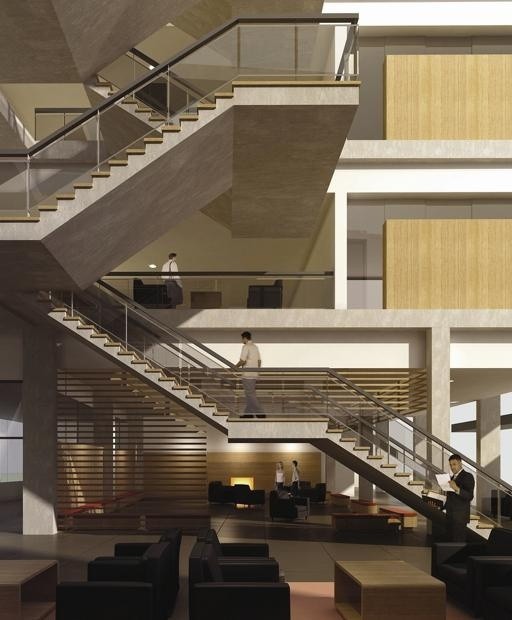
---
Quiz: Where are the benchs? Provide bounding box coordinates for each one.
[326,491,418,537]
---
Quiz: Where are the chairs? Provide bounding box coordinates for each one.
[189,530,290,619]
[270,491,297,521]
[209,481,265,510]
[432,528,512,620]
[56,526,181,620]
[133,278,171,309]
[246,280,283,308]
[291,481,326,505]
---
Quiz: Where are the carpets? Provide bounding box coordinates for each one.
[286,581,475,620]
